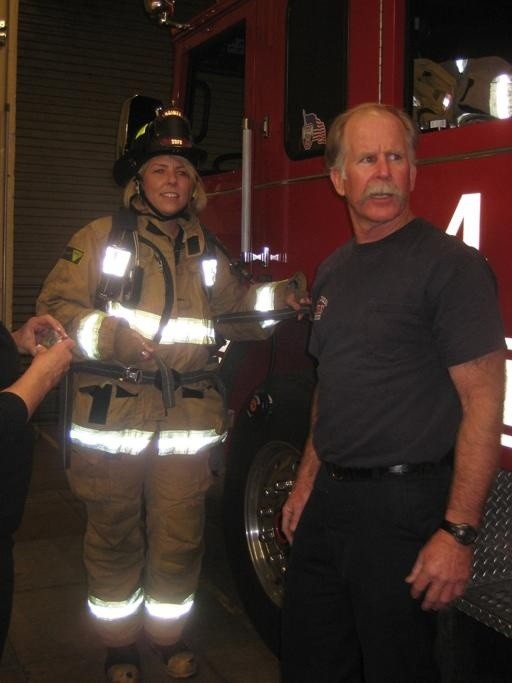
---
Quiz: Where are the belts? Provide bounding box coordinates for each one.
[325,461,440,481]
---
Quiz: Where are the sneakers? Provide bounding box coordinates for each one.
[148,639,200,679]
[105,644,141,682]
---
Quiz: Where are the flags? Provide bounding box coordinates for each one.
[306,112,327,144]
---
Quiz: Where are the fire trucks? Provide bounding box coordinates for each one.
[114,0,511,651]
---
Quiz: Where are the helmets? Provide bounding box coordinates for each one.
[134,106,207,171]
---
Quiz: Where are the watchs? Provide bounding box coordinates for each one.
[440,519,480,545]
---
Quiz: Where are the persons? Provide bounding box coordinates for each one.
[35,153,314,683]
[1,314,74,681]
[277,103,507,680]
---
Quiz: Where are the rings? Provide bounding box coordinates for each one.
[141,349,147,356]
[142,340,146,345]
